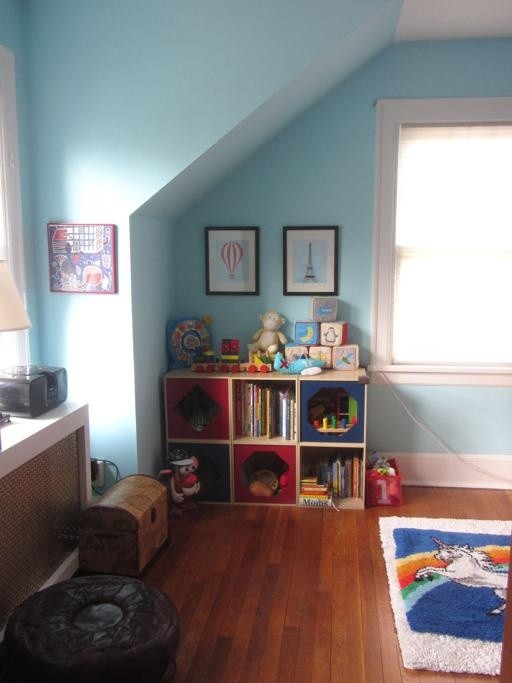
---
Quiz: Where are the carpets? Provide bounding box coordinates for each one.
[379,515,511,675]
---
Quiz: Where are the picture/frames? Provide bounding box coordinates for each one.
[282,226,339,297]
[205,225,261,296]
[47,222,117,294]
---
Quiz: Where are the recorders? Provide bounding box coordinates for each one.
[0,366,68,419]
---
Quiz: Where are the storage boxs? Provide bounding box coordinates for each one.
[365,457,402,507]
[78,475,170,572]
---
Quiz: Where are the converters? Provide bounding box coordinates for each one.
[91,458,98,481]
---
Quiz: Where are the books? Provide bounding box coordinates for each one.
[300,447,363,505]
[236,378,297,440]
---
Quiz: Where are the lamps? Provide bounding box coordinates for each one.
[0,261,32,332]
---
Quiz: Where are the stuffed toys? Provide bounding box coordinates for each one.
[273,351,325,374]
[168,447,201,513]
[246,309,288,353]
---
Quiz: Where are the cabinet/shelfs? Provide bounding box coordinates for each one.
[164,376,367,514]
